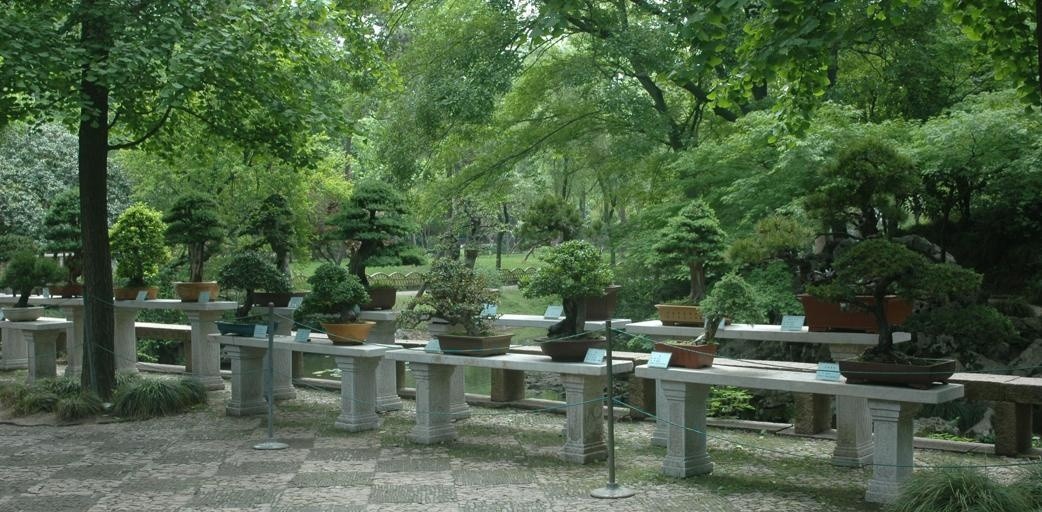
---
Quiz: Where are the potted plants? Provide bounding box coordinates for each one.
[821,234,1018,393]
[658,271,771,371]
[1,234,40,303]
[289,262,377,348]
[323,178,421,310]
[107,200,166,299]
[238,193,296,306]
[430,197,507,337]
[513,191,594,336]
[652,198,734,327]
[161,191,224,302]
[399,255,514,356]
[795,138,940,333]
[518,240,606,360]
[215,250,286,337]
[2,253,69,321]
[41,190,83,296]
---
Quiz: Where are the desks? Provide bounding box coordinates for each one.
[222,363,1042,508]
